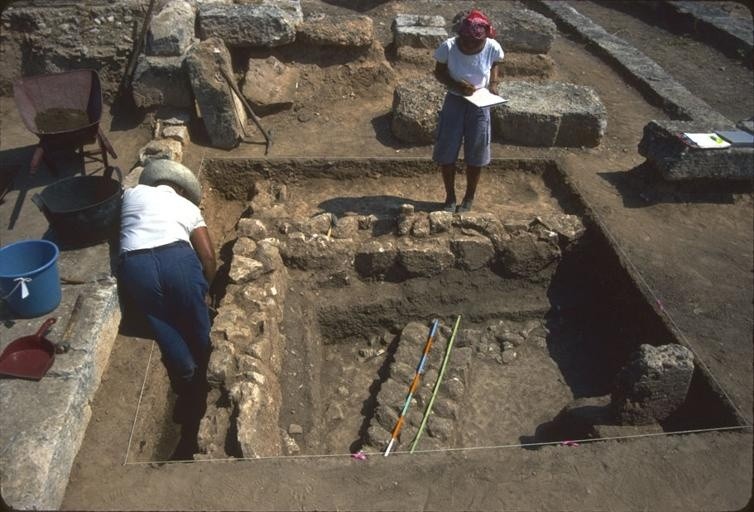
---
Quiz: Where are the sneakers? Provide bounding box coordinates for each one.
[443,196,457,212]
[459,197,473,211]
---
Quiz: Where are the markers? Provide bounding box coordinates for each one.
[709,135,723,144]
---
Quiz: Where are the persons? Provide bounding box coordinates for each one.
[432,8,509,210]
[114,158,214,418]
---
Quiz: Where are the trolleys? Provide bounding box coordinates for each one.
[13,66,122,174]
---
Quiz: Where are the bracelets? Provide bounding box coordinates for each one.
[490,79,500,84]
[445,80,453,92]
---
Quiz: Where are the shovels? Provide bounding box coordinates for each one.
[1,318,58,380]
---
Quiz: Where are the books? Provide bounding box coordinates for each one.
[714,130,753,146]
[675,132,731,151]
[462,88,508,108]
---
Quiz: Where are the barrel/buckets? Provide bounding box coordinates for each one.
[0,239,61,318]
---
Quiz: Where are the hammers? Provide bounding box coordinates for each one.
[218,63,274,155]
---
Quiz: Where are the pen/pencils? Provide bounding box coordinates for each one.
[461,79,467,84]
